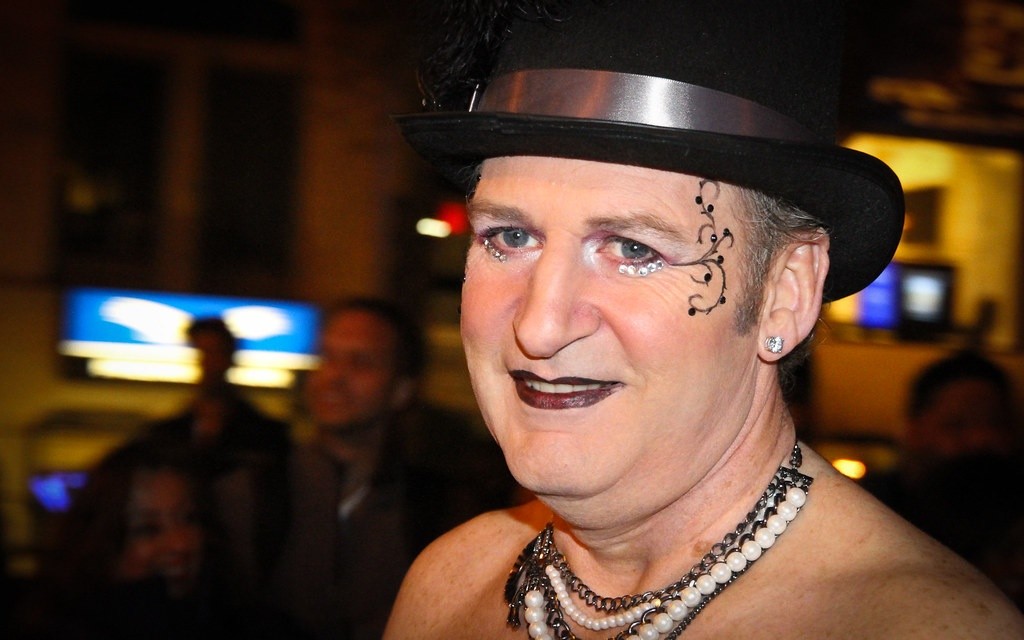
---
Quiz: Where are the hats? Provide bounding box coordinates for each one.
[395,0,907,302]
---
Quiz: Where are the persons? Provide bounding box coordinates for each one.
[12,314,290,640]
[287,294,517,640]
[851,299,1024,615]
[384,1,1024,638]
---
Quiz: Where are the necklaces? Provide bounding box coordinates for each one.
[505,441,814,640]
